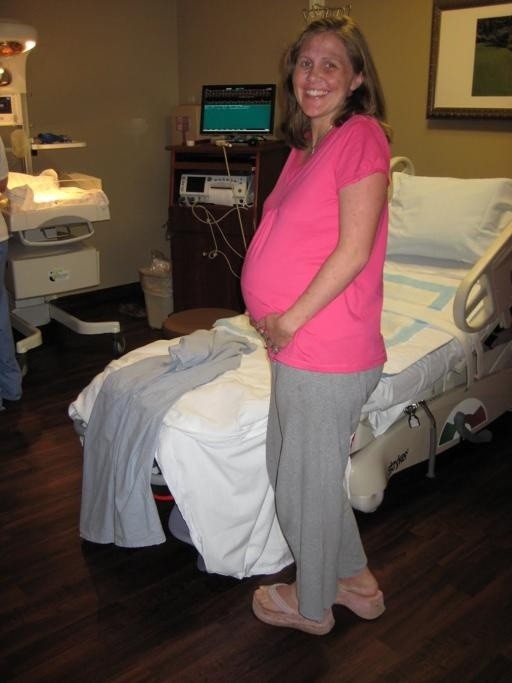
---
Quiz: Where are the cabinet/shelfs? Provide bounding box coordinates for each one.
[160,137,292,348]
[1,70,124,363]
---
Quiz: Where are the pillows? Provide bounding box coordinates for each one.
[383,171,511,267]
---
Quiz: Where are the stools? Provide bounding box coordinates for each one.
[158,304,246,351]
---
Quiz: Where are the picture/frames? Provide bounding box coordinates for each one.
[425,0,511,123]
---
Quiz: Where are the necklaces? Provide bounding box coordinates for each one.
[310,123,333,150]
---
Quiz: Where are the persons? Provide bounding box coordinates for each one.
[0,136,26,416]
[234,15,391,638]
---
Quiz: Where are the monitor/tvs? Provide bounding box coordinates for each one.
[200,83,277,143]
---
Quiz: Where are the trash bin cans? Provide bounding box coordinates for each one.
[138,267,174,330]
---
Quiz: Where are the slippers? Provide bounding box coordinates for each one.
[250,580,337,638]
[334,584,388,623]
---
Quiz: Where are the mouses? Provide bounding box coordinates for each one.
[248,137,258,145]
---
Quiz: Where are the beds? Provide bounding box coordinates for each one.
[68,196,511,526]
